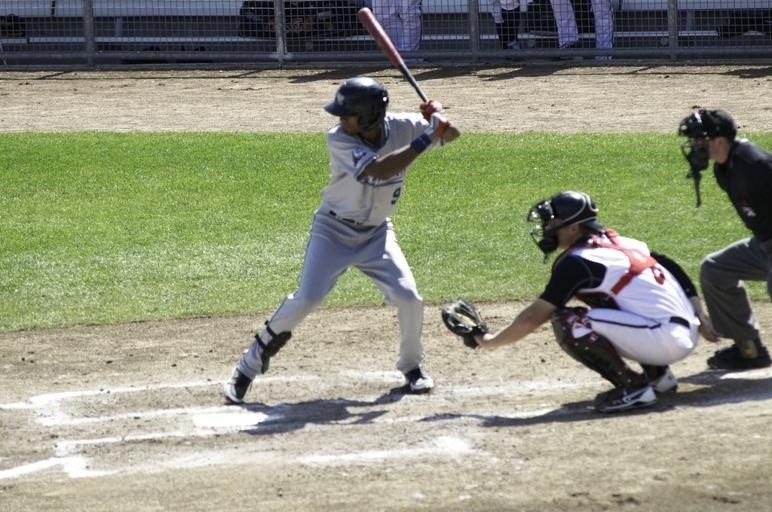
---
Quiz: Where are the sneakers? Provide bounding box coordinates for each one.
[704,340,772,371]
[640,363,679,393]
[403,366,434,393]
[222,363,254,405]
[592,373,657,415]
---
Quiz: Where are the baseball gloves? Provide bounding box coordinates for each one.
[441,298,489,349]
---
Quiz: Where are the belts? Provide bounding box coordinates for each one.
[669,313,702,332]
[328,209,360,225]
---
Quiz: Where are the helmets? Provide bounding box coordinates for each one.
[323,75,391,130]
[536,190,598,232]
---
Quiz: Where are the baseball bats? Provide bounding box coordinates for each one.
[358,6,460,143]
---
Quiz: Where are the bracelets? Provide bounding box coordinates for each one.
[411,135,432,154]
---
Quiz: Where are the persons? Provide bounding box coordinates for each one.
[439,191,720,414]
[223,77,463,404]
[550,0,616,62]
[488,0,522,61]
[676,107,771,369]
[372,1,430,65]
